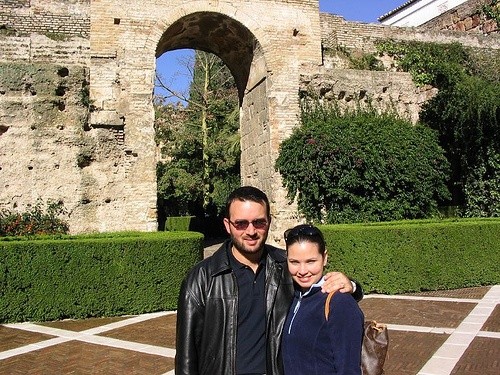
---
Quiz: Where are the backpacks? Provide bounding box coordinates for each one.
[324,291,389,375]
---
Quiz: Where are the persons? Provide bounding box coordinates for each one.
[278,224,365,375]
[175,186,363,375]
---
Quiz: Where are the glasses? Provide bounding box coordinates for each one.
[284,226,318,241]
[228,217,270,230]
